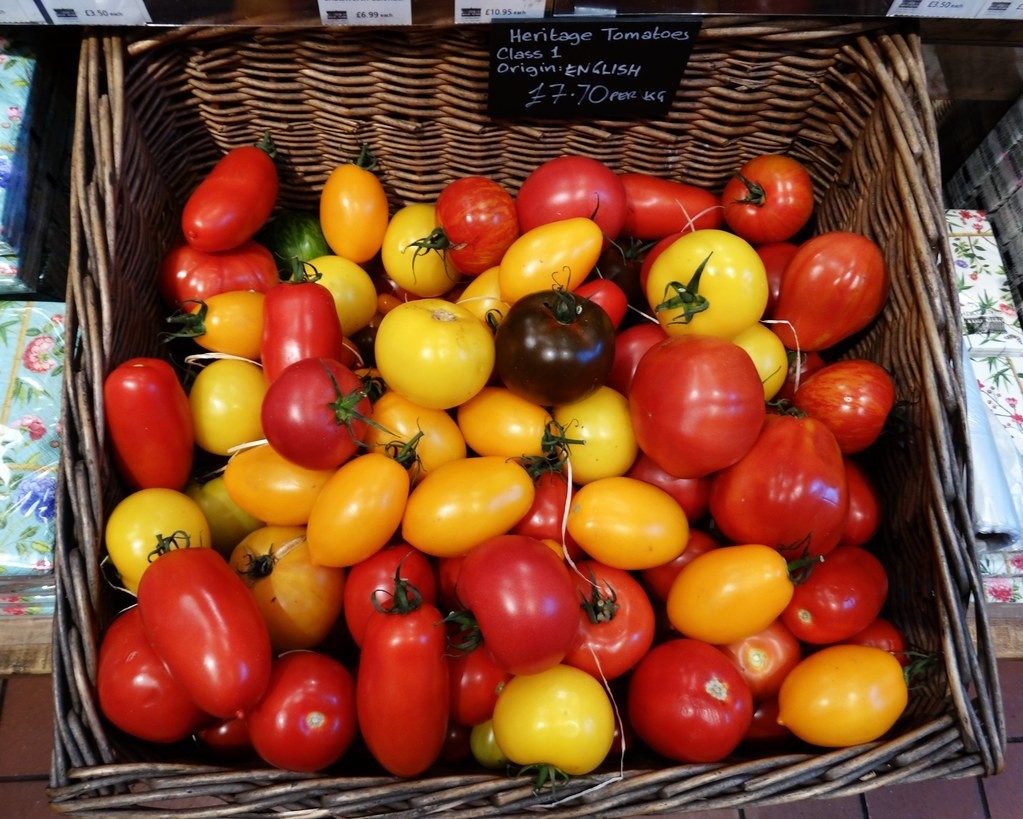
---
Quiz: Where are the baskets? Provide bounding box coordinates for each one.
[48,0,1008,819]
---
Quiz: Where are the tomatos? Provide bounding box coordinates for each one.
[95,130,936,794]
